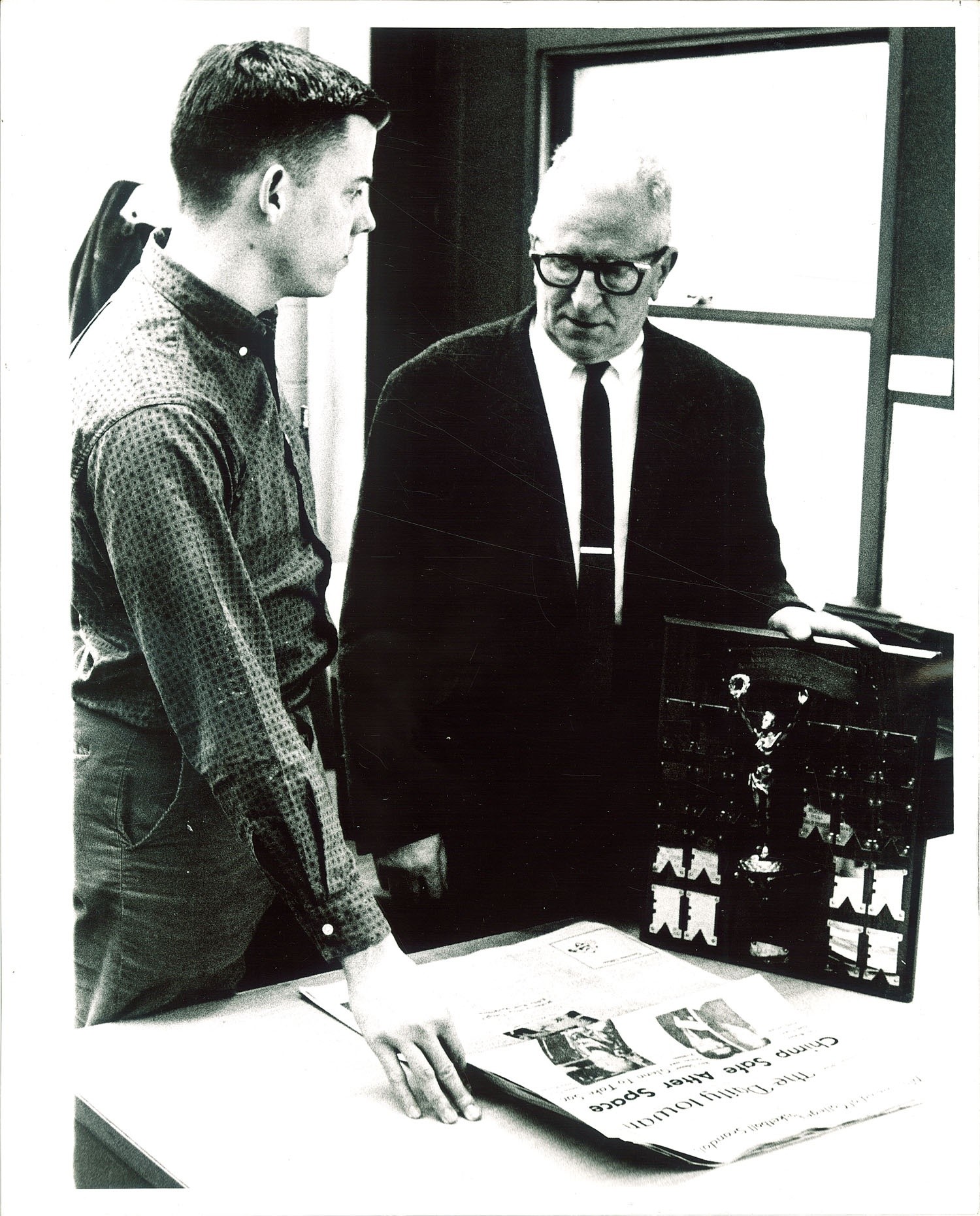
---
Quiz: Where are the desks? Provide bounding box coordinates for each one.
[74,906,980,1216]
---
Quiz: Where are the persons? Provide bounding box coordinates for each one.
[331,130,882,953]
[74,42,481,1190]
[658,998,770,1059]
[527,1016,639,1074]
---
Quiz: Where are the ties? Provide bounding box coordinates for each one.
[572,363,614,700]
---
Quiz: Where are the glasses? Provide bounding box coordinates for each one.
[530,245,667,296]
